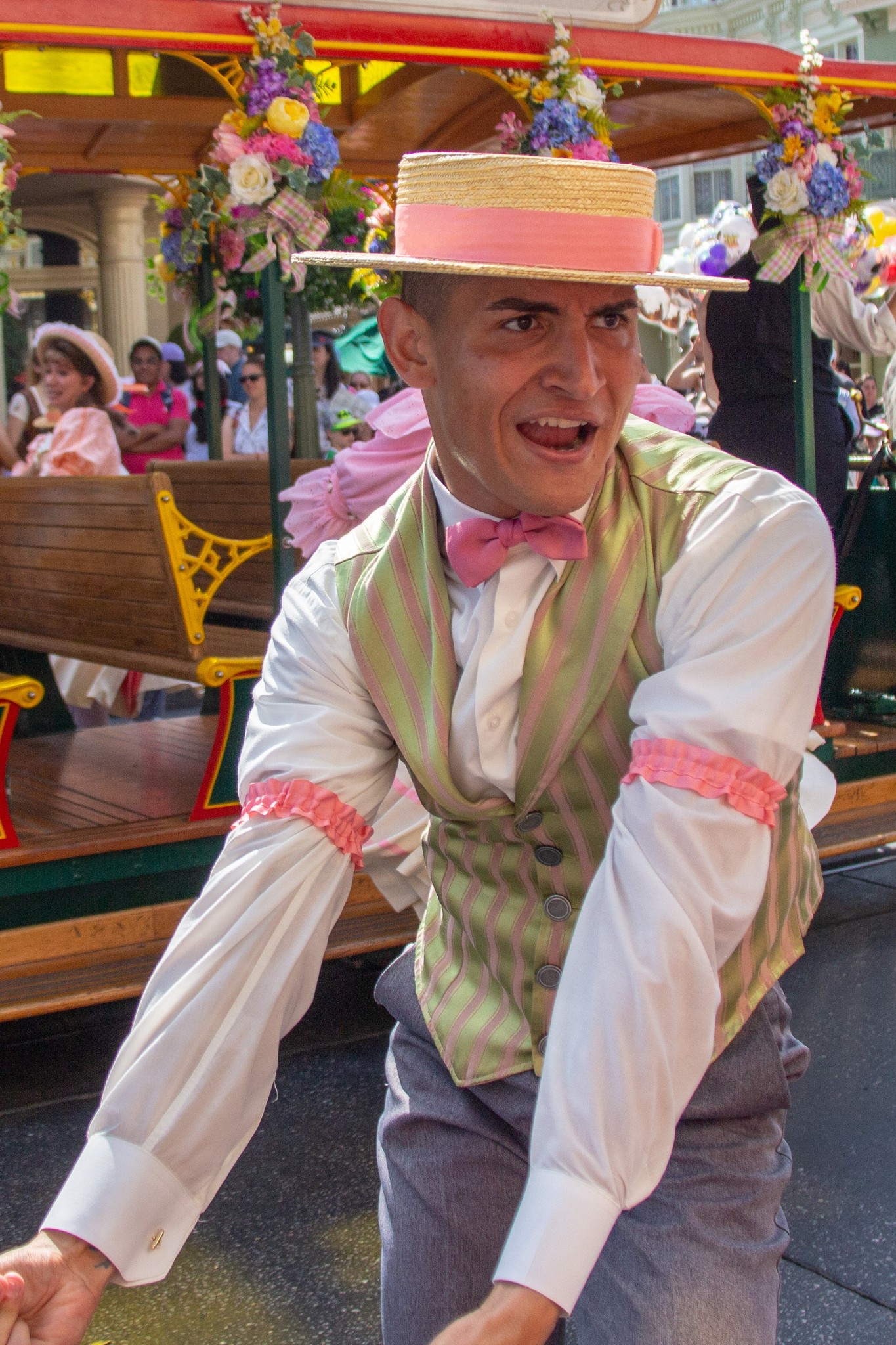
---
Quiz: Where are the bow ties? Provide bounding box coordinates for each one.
[445,510,586,588]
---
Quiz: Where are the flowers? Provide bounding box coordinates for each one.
[148,2,396,344]
[466,20,634,166]
[741,23,876,237]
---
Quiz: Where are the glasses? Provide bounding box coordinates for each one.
[342,428,359,436]
[350,381,368,390]
[239,373,265,381]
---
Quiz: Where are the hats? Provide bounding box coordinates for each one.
[689,324,699,338]
[332,408,363,431]
[309,330,332,347]
[194,360,233,378]
[746,173,767,211]
[161,342,184,361]
[129,337,163,359]
[34,321,123,409]
[217,329,242,349]
[290,151,750,292]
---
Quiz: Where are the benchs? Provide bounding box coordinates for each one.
[0,668,46,851]
[1,473,275,822]
[147,449,337,625]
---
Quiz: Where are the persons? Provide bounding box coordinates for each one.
[830,349,896,489]
[629,324,718,440]
[0,322,409,727]
[0,152,838,1345]
[696,173,896,722]
